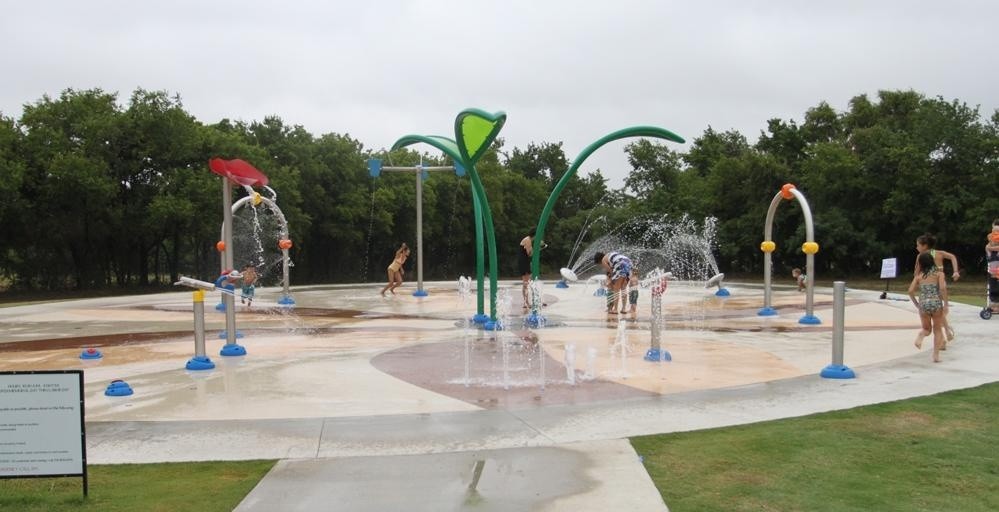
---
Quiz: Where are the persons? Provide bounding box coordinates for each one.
[381,243,410,297]
[987,225,999,258]
[605,269,616,313]
[916,232,960,350]
[984,218,999,314]
[791,268,807,291]
[594,252,633,313]
[628,267,638,312]
[518,228,536,307]
[908,253,949,363]
[240,261,258,306]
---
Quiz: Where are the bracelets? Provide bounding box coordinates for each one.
[953,270,958,272]
